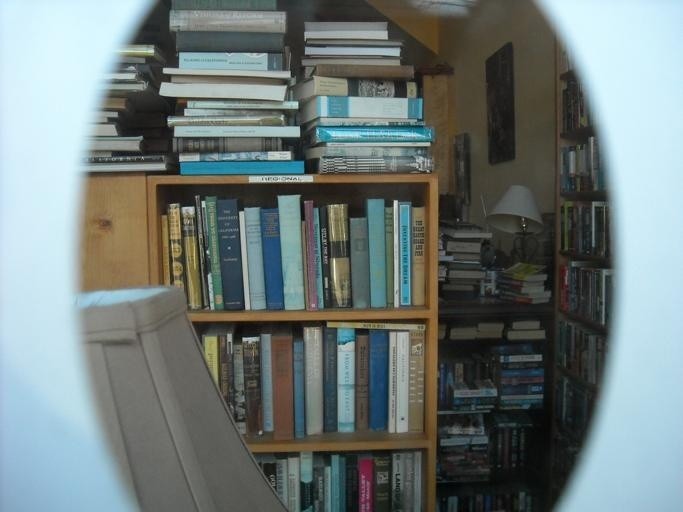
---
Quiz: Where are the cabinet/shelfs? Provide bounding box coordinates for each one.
[80,172,152,289]
[439,301,555,512]
[145,172,439,512]
[551,31,612,506]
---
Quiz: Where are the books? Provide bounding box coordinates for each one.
[188,321,426,438]
[290,21,434,173]
[554,30,614,496]
[156,2,309,174]
[436,219,550,512]
[250,450,424,511]
[159,191,426,312]
[83,44,181,175]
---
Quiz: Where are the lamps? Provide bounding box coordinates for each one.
[485,184,545,264]
